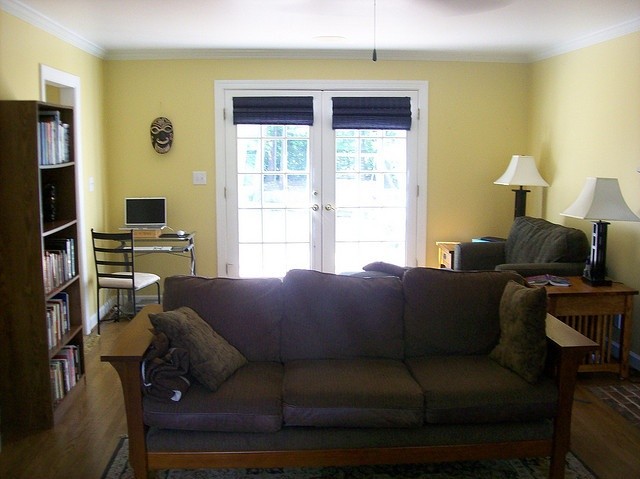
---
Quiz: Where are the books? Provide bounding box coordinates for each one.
[39,108,72,164]
[526,274,572,287]
[45,292,72,351]
[48,344,83,404]
[44,237,83,295]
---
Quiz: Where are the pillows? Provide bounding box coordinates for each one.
[364,262,408,275]
[148,308,249,391]
[488,278,547,385]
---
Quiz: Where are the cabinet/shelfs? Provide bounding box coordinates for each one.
[0,99,87,427]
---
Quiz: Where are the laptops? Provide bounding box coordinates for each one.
[118,198,167,230]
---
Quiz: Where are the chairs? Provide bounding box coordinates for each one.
[90,228,161,336]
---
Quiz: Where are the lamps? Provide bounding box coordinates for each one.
[493,154,549,217]
[559,175,640,286]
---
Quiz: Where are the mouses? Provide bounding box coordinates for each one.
[177,231,185,236]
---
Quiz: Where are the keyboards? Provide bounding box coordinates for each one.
[123,247,172,251]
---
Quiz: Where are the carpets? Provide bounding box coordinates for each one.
[101,434,598,479]
[590,380,640,427]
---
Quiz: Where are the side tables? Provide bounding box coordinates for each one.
[526,276,640,381]
[435,240,470,269]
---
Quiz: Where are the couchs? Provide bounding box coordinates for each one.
[454,215,590,274]
[99,267,598,478]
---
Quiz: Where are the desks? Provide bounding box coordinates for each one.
[114,232,197,312]
